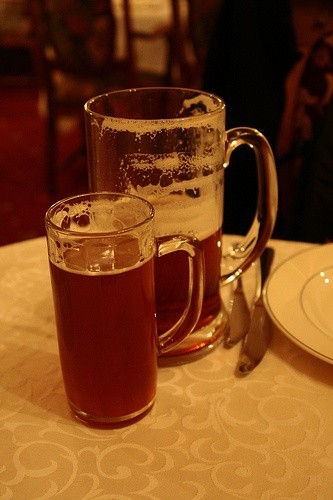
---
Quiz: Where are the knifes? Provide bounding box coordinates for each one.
[239,247,275,378]
[223,276,251,349]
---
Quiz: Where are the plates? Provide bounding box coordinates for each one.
[261,242,333,365]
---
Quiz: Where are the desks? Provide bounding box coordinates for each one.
[0,231,333,500]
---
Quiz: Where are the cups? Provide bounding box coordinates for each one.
[83,86,278,360]
[43,191,205,433]
